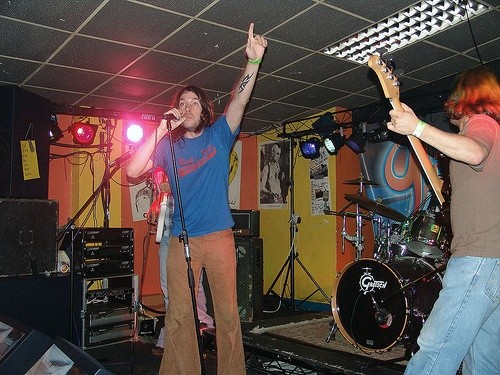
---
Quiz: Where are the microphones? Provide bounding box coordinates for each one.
[161,113,176,120]
[109,149,136,167]
[377,314,388,324]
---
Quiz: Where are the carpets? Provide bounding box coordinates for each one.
[263,317,411,361]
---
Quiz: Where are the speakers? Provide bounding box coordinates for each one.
[0,84,114,375]
[202,237,264,325]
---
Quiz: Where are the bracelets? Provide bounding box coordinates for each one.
[412,120,426,138]
[248,58,261,64]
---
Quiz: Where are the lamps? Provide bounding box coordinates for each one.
[324,132,346,155]
[367,128,391,144]
[37,118,62,145]
[298,138,322,159]
[70,121,98,146]
[312,112,338,137]
[346,131,366,155]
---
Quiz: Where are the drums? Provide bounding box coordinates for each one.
[372,222,406,258]
[405,209,454,257]
[329,253,443,355]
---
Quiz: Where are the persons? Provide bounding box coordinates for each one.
[147,166,215,357]
[387,71,500,375]
[261,144,283,203]
[127,23,268,375]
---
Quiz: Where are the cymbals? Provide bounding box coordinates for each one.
[341,192,408,223]
[341,177,381,186]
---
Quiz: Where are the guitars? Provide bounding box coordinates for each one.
[367,54,453,241]
